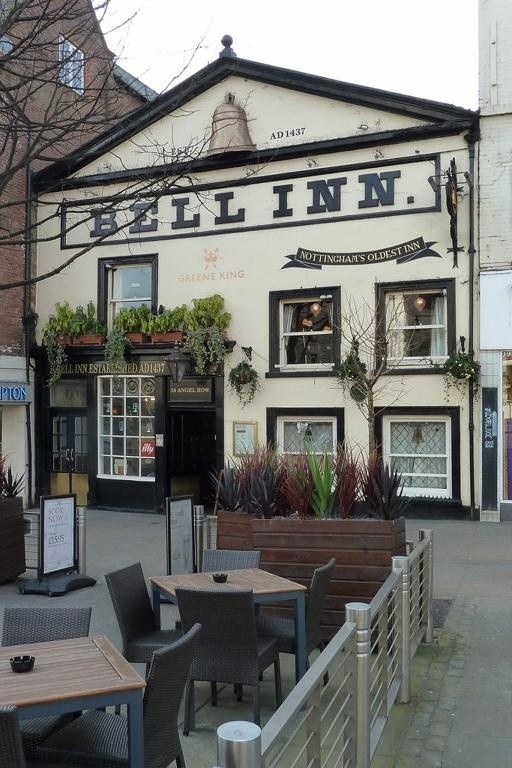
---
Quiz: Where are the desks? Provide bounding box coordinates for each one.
[148,568,308,710]
[1,633,147,768]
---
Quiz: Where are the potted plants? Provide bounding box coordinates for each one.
[149,304,186,344]
[250,446,404,646]
[72,302,104,345]
[445,350,481,411]
[106,303,149,383]
[0,464,26,585]
[217,442,250,549]
[226,359,260,408]
[184,294,232,375]
[41,301,71,388]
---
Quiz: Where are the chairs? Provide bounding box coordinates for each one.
[103,562,185,716]
[202,548,262,574]
[0,607,91,761]
[36,622,201,768]
[235,557,336,687]
[176,587,281,737]
[0,706,29,768]
